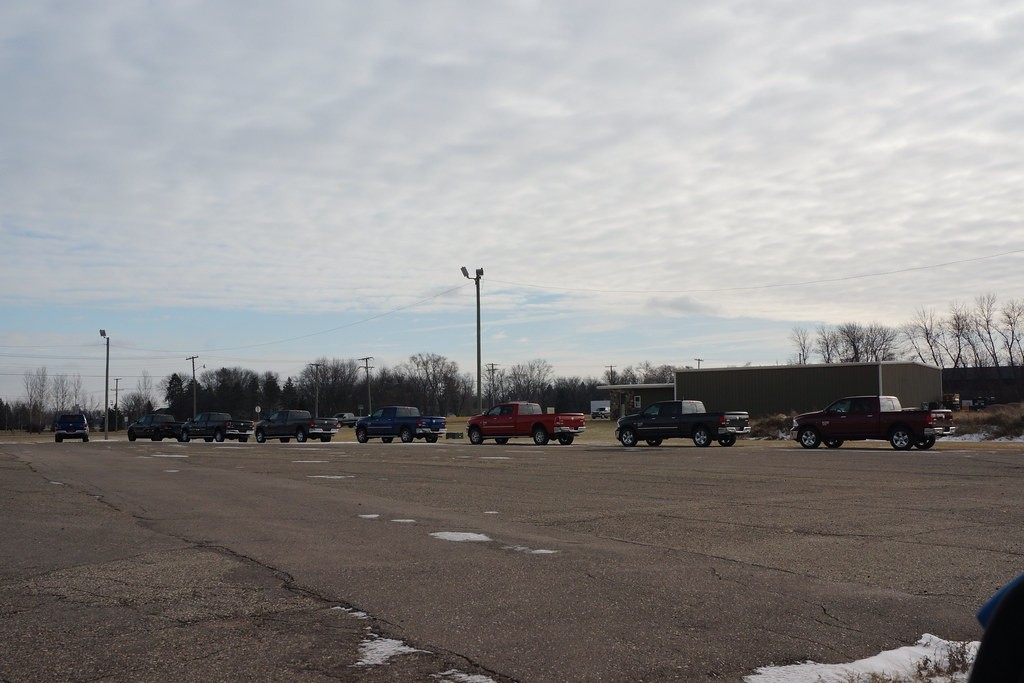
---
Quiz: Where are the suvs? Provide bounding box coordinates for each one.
[54,413,91,443]
[592,407,612,420]
[969,395,998,412]
[944,392,961,411]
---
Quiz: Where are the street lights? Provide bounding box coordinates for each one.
[460,266,486,417]
[99,329,112,440]
[185,355,206,420]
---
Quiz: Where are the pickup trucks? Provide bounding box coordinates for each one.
[356,406,448,445]
[465,402,586,446]
[127,414,185,442]
[254,410,339,444]
[180,411,255,444]
[333,413,360,428]
[789,396,957,452]
[615,399,752,448]
